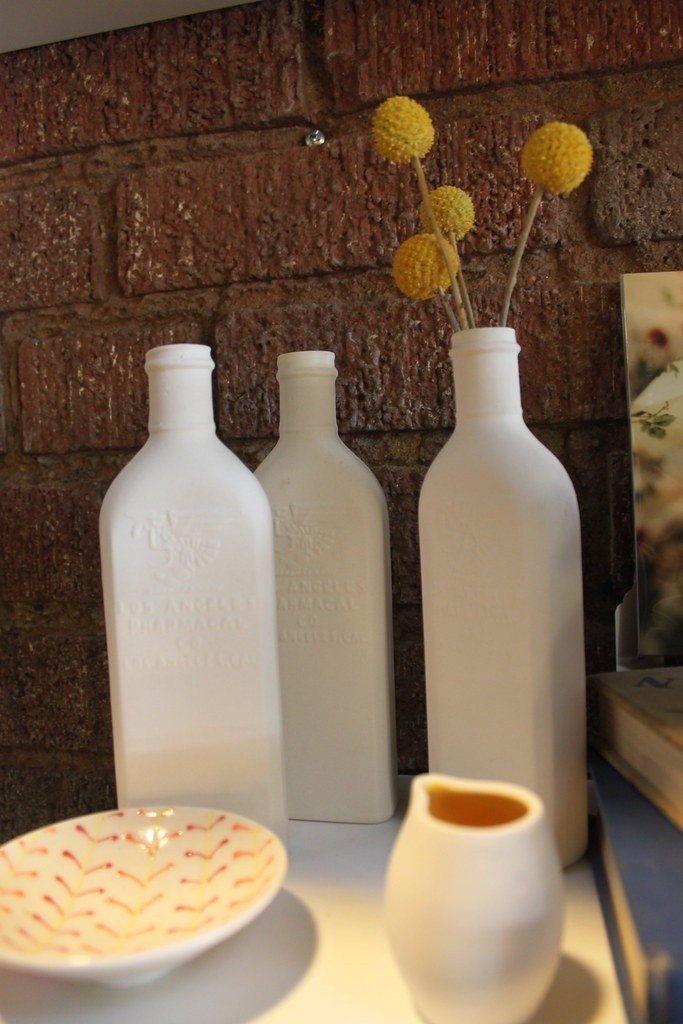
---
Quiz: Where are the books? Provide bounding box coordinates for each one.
[590,666,683,1024]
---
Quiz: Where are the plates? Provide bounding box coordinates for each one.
[0,806,287,988]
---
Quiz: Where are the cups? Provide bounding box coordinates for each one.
[386,774,565,1024]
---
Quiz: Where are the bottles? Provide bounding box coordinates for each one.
[258,348,396,823]
[99,344,284,833]
